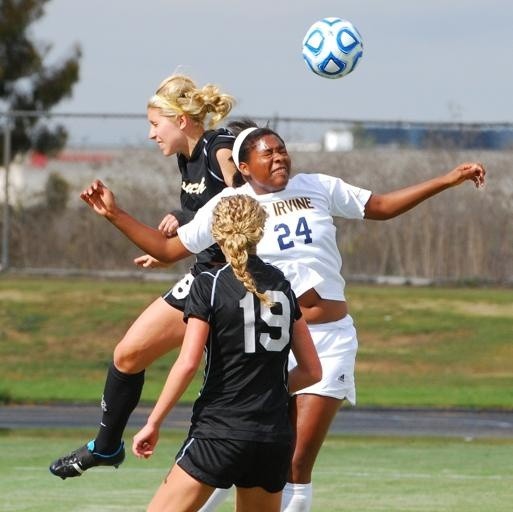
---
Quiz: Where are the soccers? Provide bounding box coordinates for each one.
[302,17,364,79]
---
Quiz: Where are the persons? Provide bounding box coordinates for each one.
[49,72,242,480]
[130,195,322,512]
[78,115,485,512]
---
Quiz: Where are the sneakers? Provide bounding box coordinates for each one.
[50,438,125,477]
